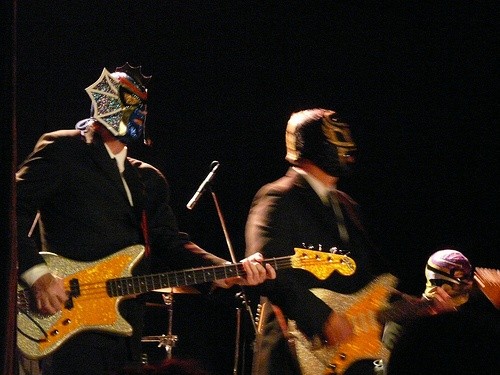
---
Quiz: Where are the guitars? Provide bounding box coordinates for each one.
[17,242,357,360]
[288,266,500,375]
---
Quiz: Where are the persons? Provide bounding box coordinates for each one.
[244,108,460,375]
[15,61,277,375]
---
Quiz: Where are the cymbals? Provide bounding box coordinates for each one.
[152,285,201,295]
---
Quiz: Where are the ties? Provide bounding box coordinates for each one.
[327,189,349,242]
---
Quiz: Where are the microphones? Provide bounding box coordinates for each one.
[186,163,220,210]
